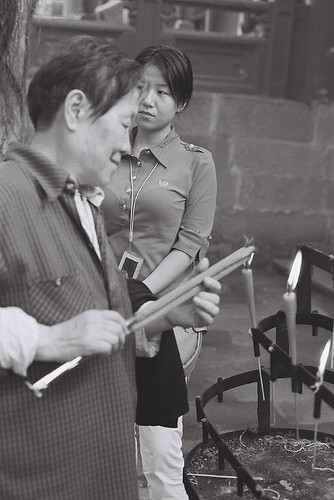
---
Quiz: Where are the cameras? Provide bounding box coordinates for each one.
[118,251,143,279]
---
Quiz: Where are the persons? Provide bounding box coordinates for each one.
[0,35,222,500]
[98,43,217,500]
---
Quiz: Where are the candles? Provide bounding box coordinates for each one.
[282,292,300,441]
[241,268,266,401]
